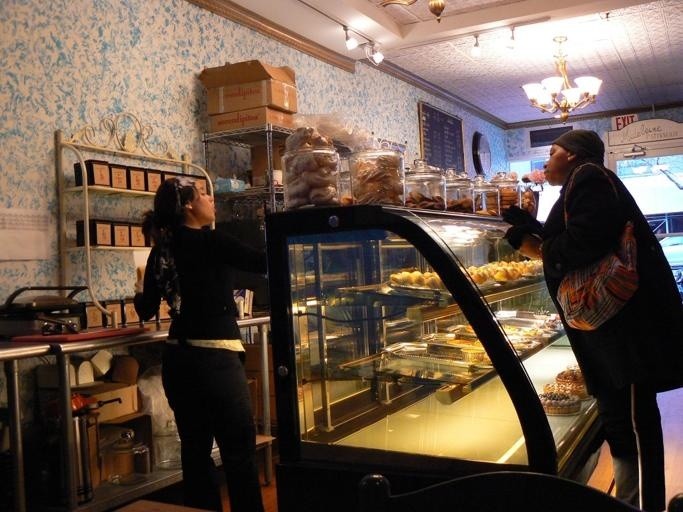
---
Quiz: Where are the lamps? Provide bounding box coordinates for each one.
[517,36,605,124]
[337,16,389,68]
[470,33,488,60]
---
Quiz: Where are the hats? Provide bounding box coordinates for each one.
[551,128,606,165]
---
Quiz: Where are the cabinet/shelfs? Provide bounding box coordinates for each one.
[267,204,616,510]
[12,307,276,512]
[48,114,217,330]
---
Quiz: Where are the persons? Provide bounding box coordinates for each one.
[498,128,681,512]
[132,177,282,510]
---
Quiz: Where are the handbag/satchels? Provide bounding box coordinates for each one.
[554,161,641,333]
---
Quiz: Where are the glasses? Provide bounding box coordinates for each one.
[178,177,198,191]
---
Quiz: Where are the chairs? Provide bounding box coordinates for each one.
[382,467,644,511]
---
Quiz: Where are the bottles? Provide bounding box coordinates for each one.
[279,125,539,222]
[100,418,182,486]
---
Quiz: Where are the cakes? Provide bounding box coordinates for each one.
[538,369,587,414]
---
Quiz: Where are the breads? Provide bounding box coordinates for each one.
[390,261,543,288]
[283,126,535,216]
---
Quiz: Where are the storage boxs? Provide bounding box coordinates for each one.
[212,107,295,131]
[196,57,295,114]
[248,143,285,182]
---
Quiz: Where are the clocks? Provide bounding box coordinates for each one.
[470,131,491,177]
